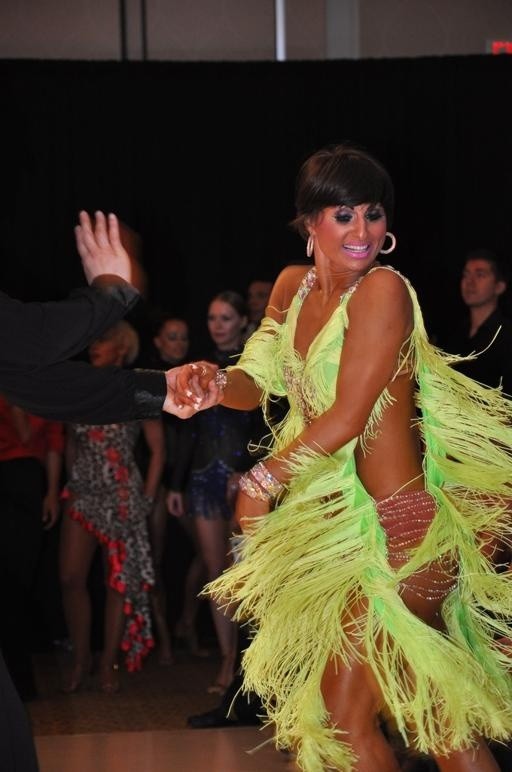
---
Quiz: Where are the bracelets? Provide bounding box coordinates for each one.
[236,471,274,507]
[248,461,284,500]
[215,369,228,390]
[142,494,156,504]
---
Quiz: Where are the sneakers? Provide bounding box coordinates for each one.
[189,708,254,727]
[51,638,72,652]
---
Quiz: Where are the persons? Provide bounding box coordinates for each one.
[0,208,227,772]
[52,322,169,696]
[242,272,278,326]
[175,138,512,771]
[162,277,277,697]
[0,400,64,689]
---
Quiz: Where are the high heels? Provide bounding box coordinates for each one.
[174,620,211,657]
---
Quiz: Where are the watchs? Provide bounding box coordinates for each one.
[141,313,219,675]
[446,248,512,399]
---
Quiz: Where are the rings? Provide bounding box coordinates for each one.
[198,365,208,377]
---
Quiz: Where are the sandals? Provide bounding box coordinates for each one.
[207,656,233,694]
[61,657,122,695]
